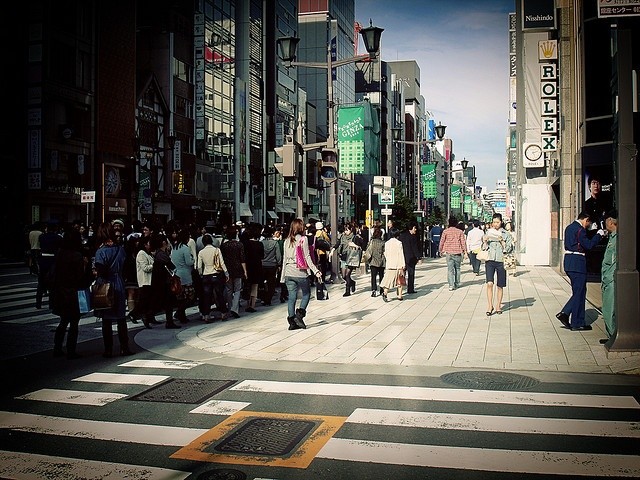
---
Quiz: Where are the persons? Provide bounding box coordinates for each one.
[399,224,419,294]
[479,222,486,233]
[49,252,80,351]
[324,227,332,238]
[113,218,126,230]
[316,222,330,282]
[282,219,323,332]
[439,216,467,291]
[151,235,183,330]
[556,212,604,331]
[28,220,43,278]
[141,224,153,237]
[273,228,282,241]
[503,221,516,277]
[382,228,406,302]
[92,222,131,343]
[467,220,484,276]
[305,223,316,265]
[424,225,430,258]
[200,226,207,234]
[329,223,366,298]
[429,221,435,231]
[64,219,81,252]
[259,228,282,306]
[44,225,49,234]
[386,219,396,229]
[430,221,443,258]
[367,228,385,297]
[79,225,88,235]
[242,225,265,312]
[172,230,194,323]
[114,229,123,244]
[585,176,613,211]
[197,235,229,321]
[599,214,618,343]
[485,223,490,229]
[369,218,385,240]
[220,228,248,318]
[129,238,155,330]
[171,230,178,239]
[483,213,511,316]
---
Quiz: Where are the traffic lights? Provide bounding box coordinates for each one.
[274,145,299,182]
[321,147,338,184]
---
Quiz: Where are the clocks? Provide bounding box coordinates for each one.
[525,145,543,161]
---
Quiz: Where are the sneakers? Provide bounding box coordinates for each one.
[371,290,376,296]
[280,297,285,303]
[206,315,215,321]
[221,314,229,320]
[449,286,456,290]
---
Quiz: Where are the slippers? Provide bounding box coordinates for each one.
[486,306,494,315]
[497,311,502,314]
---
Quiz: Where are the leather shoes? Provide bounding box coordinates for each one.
[571,325,592,331]
[142,317,152,329]
[151,317,162,323]
[245,307,256,312]
[166,321,182,329]
[556,312,571,327]
[231,310,239,317]
[175,310,187,323]
[102,349,113,357]
[397,297,403,300]
[128,312,138,323]
[382,292,387,301]
[120,347,136,355]
[409,290,417,293]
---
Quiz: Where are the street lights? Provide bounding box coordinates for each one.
[391,121,447,212]
[275,18,385,284]
[431,157,469,228]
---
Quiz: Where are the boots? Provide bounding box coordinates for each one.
[350,279,356,292]
[287,314,298,330]
[294,308,306,328]
[343,286,350,296]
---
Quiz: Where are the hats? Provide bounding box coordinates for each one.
[315,222,323,229]
[236,221,245,226]
[112,220,124,228]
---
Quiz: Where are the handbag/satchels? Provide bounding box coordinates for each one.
[316,273,329,300]
[214,249,223,272]
[503,251,516,269]
[296,236,315,269]
[363,240,373,263]
[168,273,182,294]
[476,242,490,261]
[346,244,362,268]
[89,245,122,310]
[397,274,406,285]
